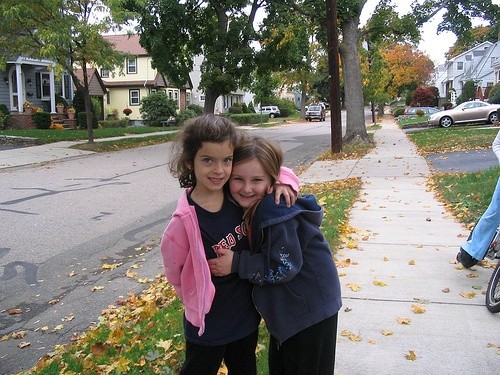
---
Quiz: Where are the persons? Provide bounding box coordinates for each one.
[159,115,300,375]
[457,129,500,268]
[206,135,342,375]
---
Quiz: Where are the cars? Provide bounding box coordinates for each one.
[404,107,440,115]
[427,100,500,128]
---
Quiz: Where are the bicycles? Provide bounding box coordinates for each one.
[456,218,500,313]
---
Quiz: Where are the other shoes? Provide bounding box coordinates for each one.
[458,250,478,269]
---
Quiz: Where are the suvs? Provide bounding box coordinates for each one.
[257,106,281,119]
[305,102,326,122]
